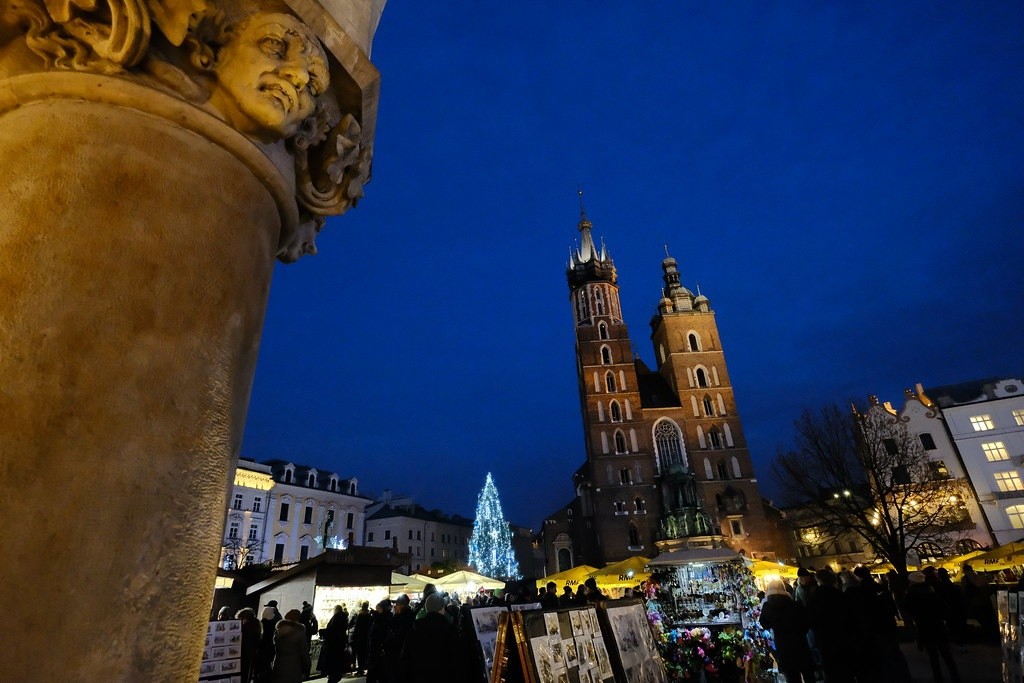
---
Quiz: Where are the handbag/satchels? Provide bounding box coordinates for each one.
[345,650,350,663]
[914,640,923,652]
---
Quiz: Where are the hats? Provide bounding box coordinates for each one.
[797,568,809,576]
[424,584,435,599]
[380,599,390,612]
[285,609,300,620]
[264,600,277,607]
[391,594,410,604]
[908,572,925,582]
[235,608,255,619]
[426,595,444,612]
[302,601,311,611]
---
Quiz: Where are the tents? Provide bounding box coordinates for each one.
[389,538,1024,603]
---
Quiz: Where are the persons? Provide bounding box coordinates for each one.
[755,563,1005,683]
[0,0,374,261]
[218,581,648,683]
